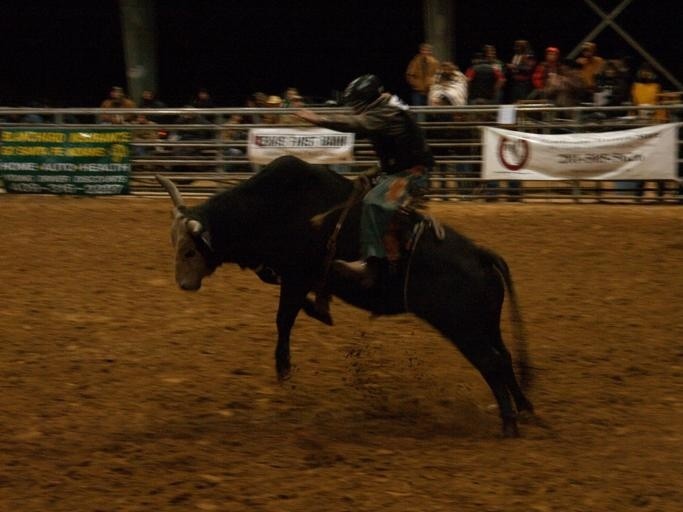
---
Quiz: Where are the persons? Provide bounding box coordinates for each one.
[293,75,434,284]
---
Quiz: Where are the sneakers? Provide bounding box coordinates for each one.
[335,259,375,282]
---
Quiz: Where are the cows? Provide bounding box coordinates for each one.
[153,153,537,440]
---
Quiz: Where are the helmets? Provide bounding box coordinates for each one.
[344,74,385,108]
